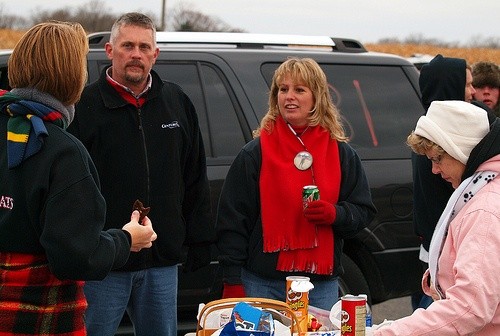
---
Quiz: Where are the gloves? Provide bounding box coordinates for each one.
[303,199,336,225]
[222,285,245,298]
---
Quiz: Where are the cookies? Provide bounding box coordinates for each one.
[133,200,150,219]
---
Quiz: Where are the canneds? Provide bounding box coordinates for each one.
[303,185,320,211]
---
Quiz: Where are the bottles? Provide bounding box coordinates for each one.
[359,294,371,328]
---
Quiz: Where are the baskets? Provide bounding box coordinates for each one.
[196,298,302,336]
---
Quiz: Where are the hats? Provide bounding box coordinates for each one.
[414,100,490,166]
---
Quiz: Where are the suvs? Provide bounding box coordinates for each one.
[0,32,455,336]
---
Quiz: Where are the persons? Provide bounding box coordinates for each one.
[411,54,476,311]
[0,20,157,336]
[67,13,212,336]
[215,58,378,312]
[363,101,500,336]
[471,62,500,118]
